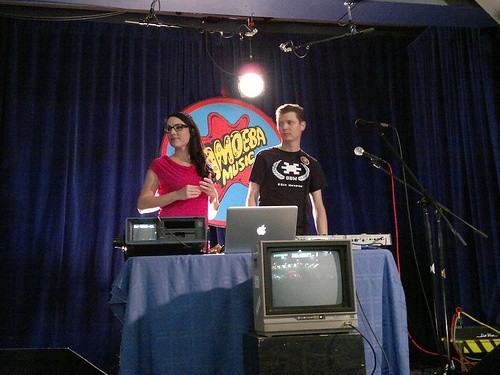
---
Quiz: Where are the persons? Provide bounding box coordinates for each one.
[247,104,328,236]
[137,113,220,252]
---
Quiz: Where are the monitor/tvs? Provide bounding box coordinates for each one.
[252,239,358,336]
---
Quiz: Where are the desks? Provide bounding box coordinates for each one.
[108,249,411,375]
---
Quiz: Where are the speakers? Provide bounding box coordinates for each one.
[451,325,500,375]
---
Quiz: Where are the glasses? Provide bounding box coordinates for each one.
[164,124,187,133]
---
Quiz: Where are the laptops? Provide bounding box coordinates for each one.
[224,205,299,254]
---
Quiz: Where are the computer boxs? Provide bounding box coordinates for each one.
[123,217,208,255]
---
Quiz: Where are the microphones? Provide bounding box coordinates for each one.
[355,120,389,128]
[354,147,385,163]
